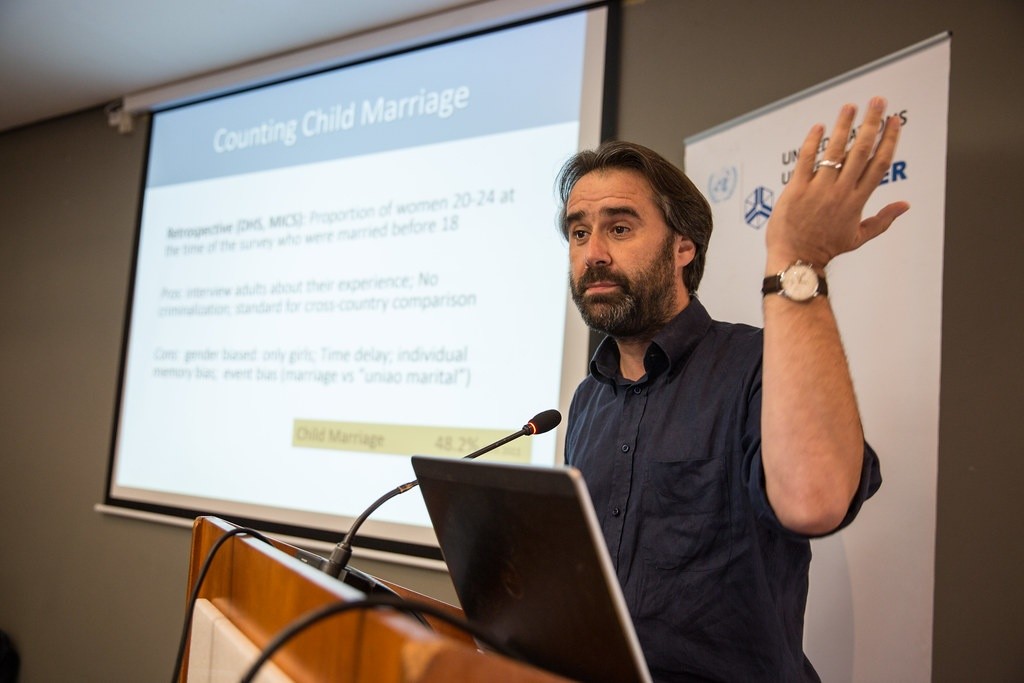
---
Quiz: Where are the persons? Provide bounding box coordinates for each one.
[557,96,909,683]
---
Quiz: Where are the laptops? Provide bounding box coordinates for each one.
[411,455,653,683]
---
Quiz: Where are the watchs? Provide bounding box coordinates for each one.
[762,260,828,303]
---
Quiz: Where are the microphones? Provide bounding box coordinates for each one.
[297,409,562,597]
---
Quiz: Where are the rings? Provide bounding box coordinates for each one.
[815,160,843,170]
